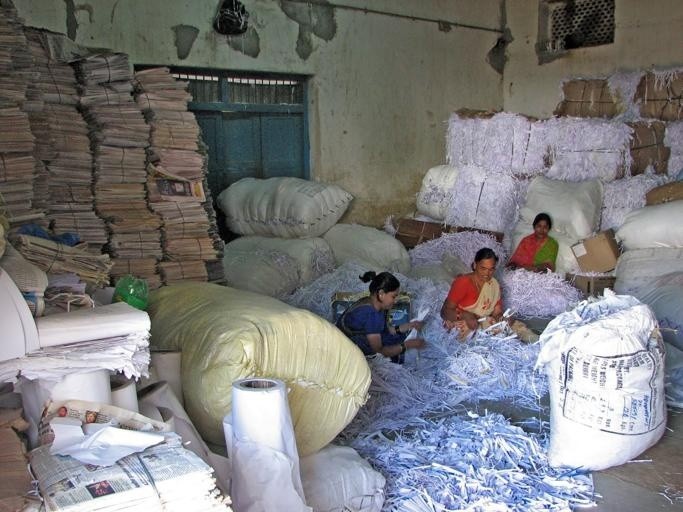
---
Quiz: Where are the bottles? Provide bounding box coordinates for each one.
[110,272,148,312]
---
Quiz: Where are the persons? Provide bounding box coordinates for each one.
[441,248,540,345]
[510,213,559,274]
[336,270,424,363]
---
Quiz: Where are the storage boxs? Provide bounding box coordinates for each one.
[570,229,620,274]
[565,273,616,300]
